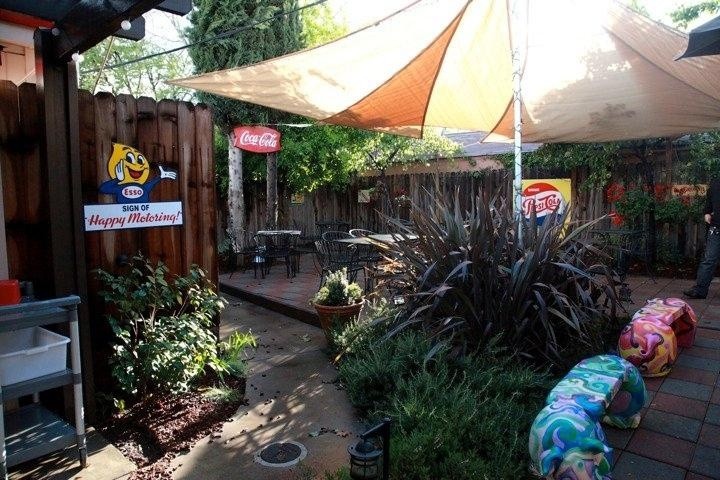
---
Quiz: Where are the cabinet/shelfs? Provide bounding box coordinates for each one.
[0,294,87,480]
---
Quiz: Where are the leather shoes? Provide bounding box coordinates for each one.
[683,290,705,299]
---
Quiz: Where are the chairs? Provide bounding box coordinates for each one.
[229,221,385,292]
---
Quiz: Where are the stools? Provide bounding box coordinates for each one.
[528,355,652,480]
[617,296,699,378]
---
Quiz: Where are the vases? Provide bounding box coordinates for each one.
[315,305,361,343]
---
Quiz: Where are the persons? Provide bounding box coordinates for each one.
[681,171,720,300]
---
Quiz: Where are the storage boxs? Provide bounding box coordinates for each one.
[0,326,72,386]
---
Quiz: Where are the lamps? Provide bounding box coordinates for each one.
[347,418,390,480]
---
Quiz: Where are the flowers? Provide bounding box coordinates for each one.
[310,268,363,305]
